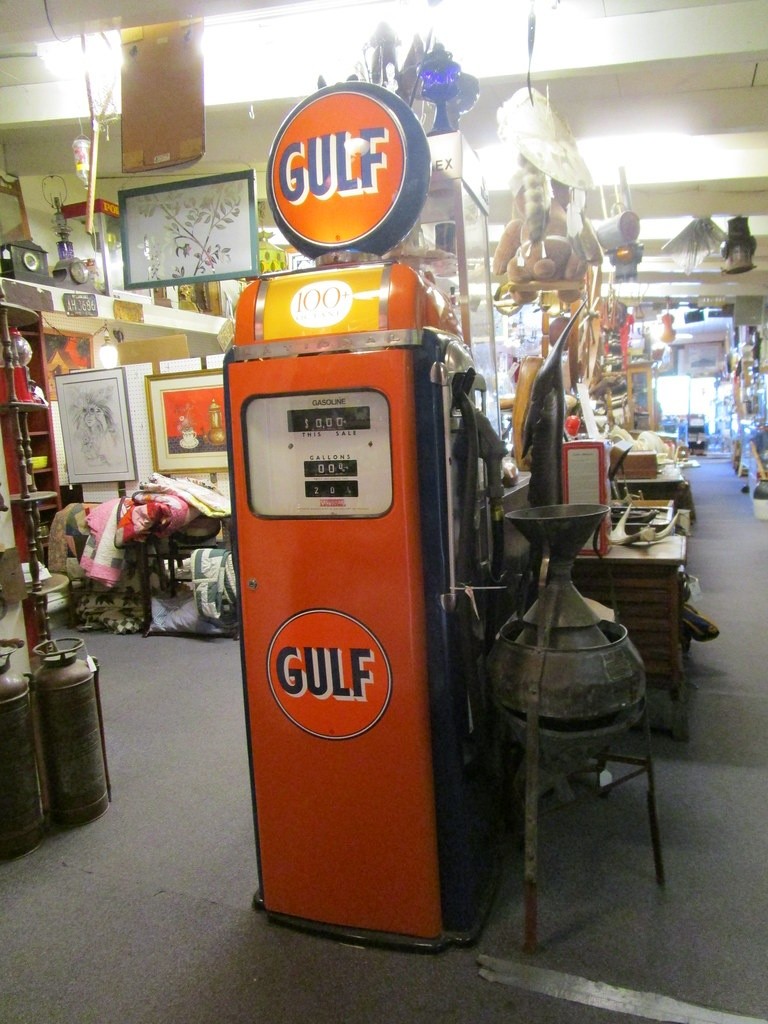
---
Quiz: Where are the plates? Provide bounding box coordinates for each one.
[179,438,199,449]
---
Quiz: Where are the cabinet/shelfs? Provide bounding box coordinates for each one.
[0,311,62,569]
[574,534,690,742]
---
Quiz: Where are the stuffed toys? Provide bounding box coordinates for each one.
[493,150,589,304]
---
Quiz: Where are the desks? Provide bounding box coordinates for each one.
[617,464,683,501]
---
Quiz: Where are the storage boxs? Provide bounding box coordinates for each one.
[561,438,610,557]
[616,449,657,479]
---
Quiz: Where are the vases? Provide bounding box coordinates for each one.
[753,478,768,520]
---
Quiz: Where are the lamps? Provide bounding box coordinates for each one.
[94,320,119,370]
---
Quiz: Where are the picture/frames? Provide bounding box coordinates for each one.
[117,169,260,291]
[53,367,139,484]
[44,327,95,401]
[143,368,228,474]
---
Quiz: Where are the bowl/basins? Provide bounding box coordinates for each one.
[30,456,48,470]
